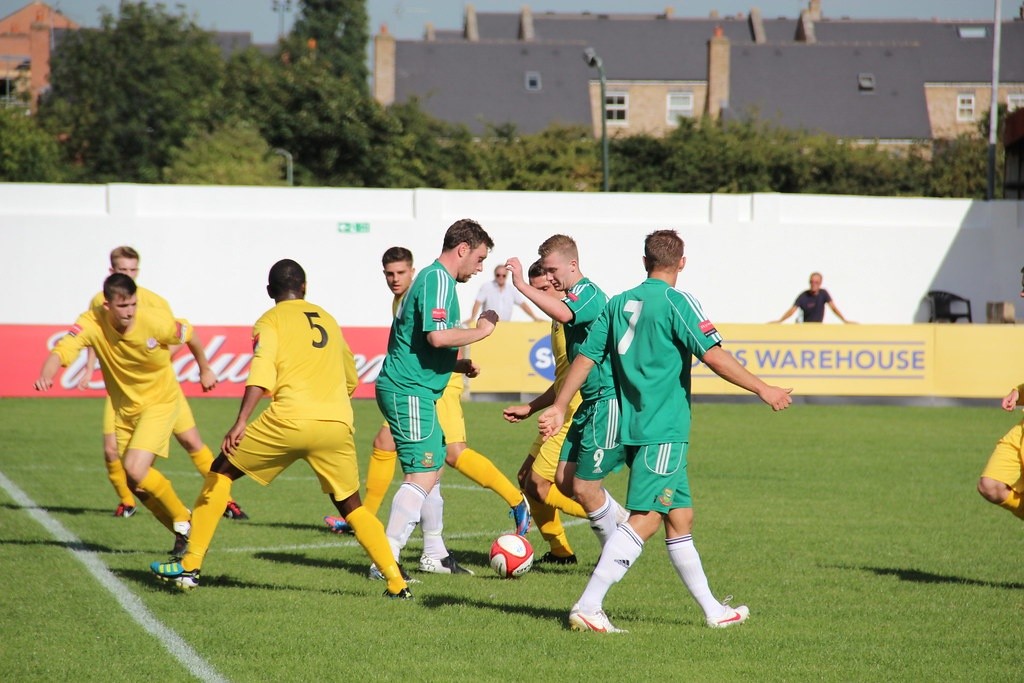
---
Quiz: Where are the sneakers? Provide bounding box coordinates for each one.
[369,561,423,583]
[151,556,199,589]
[568,602,629,633]
[323,515,355,536]
[708,604,750,628]
[508,491,532,539]
[419,553,475,573]
[532,551,578,565]
[222,502,249,520]
[383,587,413,599]
[167,513,193,555]
[114,503,136,517]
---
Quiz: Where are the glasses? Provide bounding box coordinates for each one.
[496,273,507,277]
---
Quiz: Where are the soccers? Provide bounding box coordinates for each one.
[489,534,535,577]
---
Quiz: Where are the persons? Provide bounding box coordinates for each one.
[34,272,218,556]
[500,235,632,569]
[367,218,500,583]
[76,247,249,521]
[977,265,1024,521]
[766,272,859,323]
[147,257,415,599]
[464,264,546,322]
[323,246,532,537]
[538,231,794,632]
